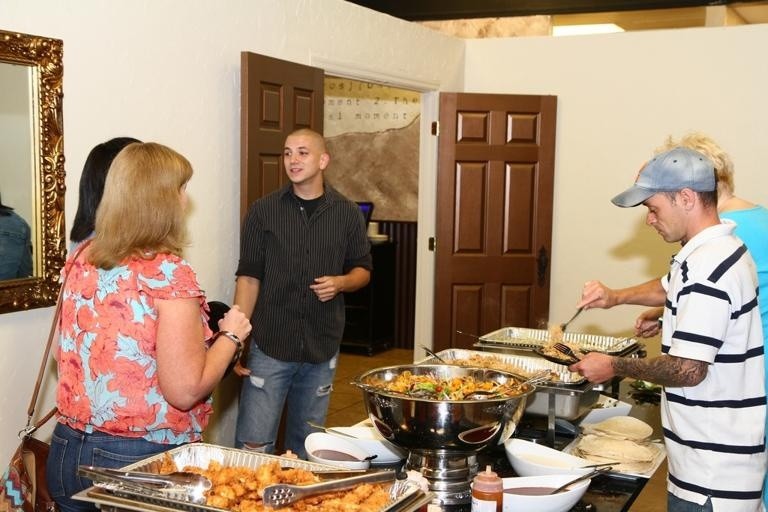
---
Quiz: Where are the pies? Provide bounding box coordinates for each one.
[571,416,661,474]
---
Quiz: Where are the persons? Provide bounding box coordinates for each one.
[46,142,256,511]
[568,146,767,512]
[233,129,373,463]
[69,136,144,254]
[0,189,34,279]
[630,133,767,511]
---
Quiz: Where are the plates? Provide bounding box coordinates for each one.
[561,432,667,481]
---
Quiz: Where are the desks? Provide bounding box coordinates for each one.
[485,380,665,511]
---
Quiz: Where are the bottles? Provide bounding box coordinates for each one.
[471,465,503,512]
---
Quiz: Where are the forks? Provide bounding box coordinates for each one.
[553,343,581,362]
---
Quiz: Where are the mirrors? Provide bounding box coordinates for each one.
[0,29,67,314]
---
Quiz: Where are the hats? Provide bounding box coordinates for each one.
[611,147,718,212]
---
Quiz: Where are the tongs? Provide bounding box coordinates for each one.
[76,464,212,505]
[262,462,405,510]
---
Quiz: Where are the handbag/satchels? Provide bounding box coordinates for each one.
[0,416,56,512]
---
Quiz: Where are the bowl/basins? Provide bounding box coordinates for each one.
[570,393,633,427]
[470,474,592,512]
[304,432,370,469]
[504,438,596,477]
[325,426,409,463]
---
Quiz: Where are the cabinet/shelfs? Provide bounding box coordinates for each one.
[339,242,397,356]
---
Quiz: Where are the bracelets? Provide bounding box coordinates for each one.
[210,330,242,365]
